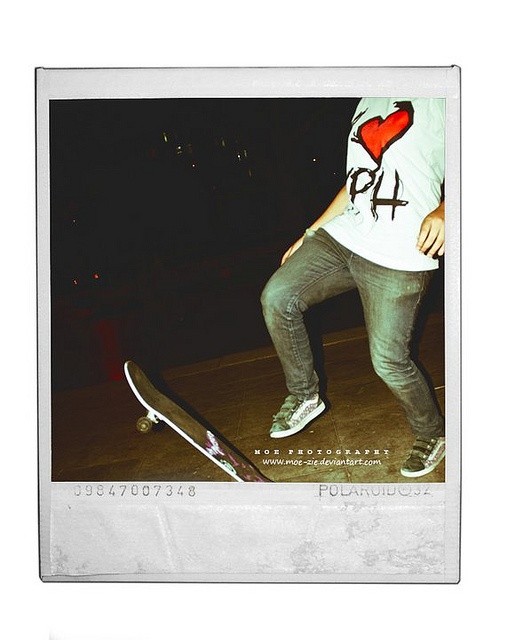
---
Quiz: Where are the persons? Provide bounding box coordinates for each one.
[259,97,447,476]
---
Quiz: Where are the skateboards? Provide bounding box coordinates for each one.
[124,360,273,482]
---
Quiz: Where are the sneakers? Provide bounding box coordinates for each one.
[269,393,326,438]
[400,436,445,478]
